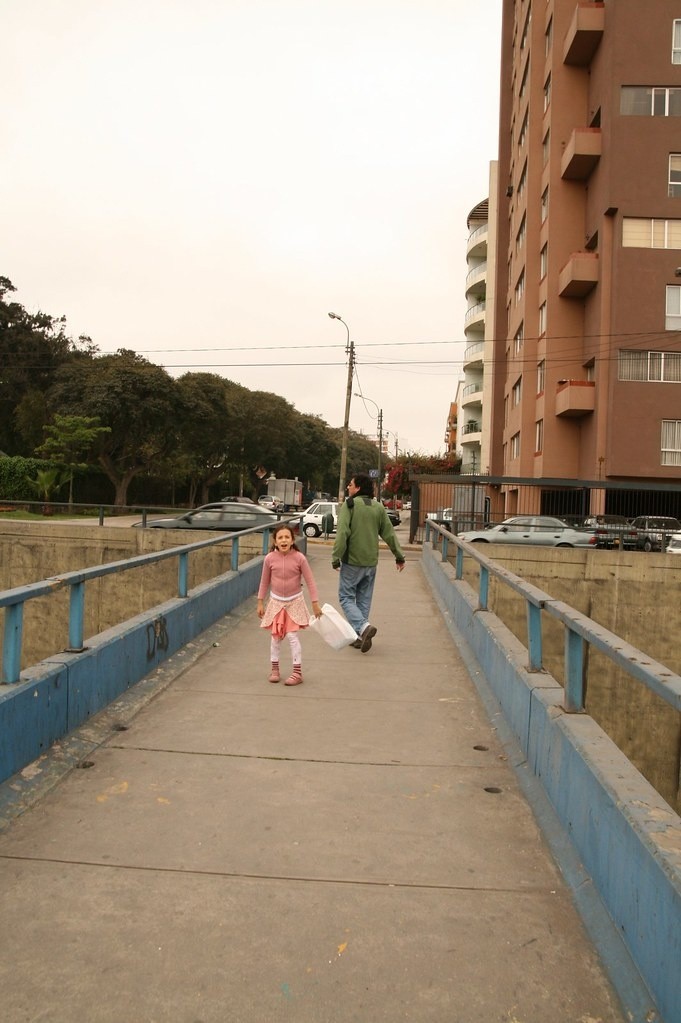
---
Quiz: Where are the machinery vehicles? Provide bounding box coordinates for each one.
[312,492,338,504]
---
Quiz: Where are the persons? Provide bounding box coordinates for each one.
[256,523,322,686]
[331,474,406,653]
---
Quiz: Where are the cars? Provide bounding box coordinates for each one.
[631,516,681,552]
[221,497,254,504]
[423,508,453,536]
[457,516,597,549]
[402,502,411,510]
[387,510,401,527]
[383,500,402,509]
[575,515,638,547]
[131,502,301,536]
[289,502,339,537]
[666,534,681,553]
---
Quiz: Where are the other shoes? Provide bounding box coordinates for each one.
[285,676,303,686]
[269,672,279,682]
[350,639,362,648]
[361,625,377,653]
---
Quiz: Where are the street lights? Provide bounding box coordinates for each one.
[377,427,398,466]
[328,312,356,502]
[354,393,382,502]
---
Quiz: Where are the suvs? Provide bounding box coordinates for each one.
[257,495,285,513]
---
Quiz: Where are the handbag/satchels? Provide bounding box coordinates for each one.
[310,603,359,651]
